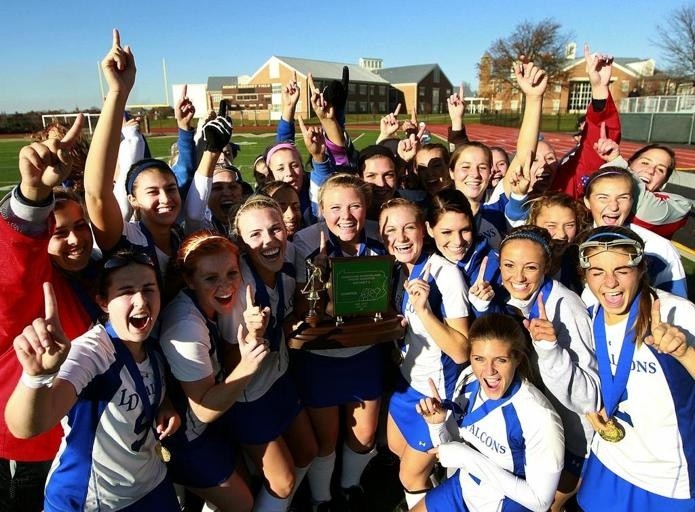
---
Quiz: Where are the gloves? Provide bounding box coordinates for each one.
[320,66,350,126]
[202,97,233,154]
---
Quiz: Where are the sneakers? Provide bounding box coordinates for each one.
[337,483,372,512]
